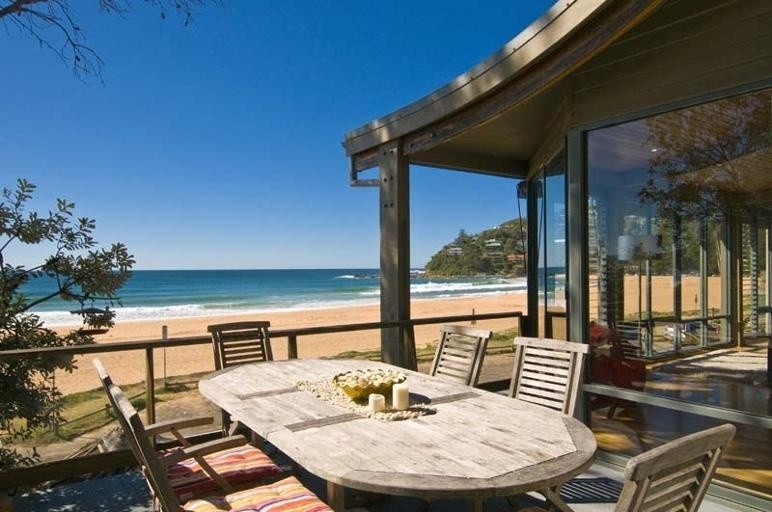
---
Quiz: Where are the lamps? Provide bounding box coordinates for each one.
[618,235,661,356]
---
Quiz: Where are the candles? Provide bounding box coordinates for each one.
[392,383,409,410]
[368,393,385,412]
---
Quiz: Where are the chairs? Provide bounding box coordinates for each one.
[427,323,494,390]
[507,337,592,419]
[205,322,275,440]
[106,383,339,512]
[93,357,284,512]
[507,421,734,511]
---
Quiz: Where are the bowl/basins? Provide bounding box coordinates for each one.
[334,368,409,402]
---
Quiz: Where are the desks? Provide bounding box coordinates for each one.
[197,358,598,512]
